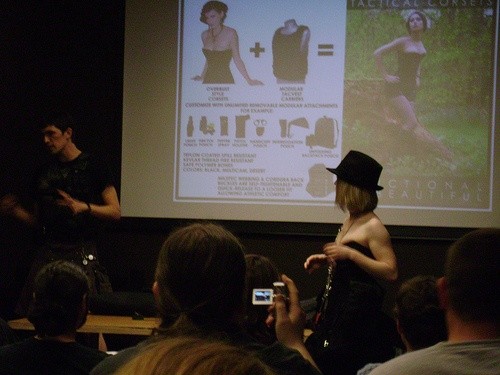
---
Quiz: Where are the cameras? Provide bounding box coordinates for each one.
[252,282,288,306]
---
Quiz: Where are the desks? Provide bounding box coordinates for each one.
[8,312,162,351]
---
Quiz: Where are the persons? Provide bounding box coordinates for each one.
[0,111,121,314]
[374,10,428,154]
[191,0,262,85]
[271,19,311,84]
[0,260,111,375]
[89,151,500,375]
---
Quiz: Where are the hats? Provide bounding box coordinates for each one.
[325,150,385,192]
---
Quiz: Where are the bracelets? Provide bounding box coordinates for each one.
[87,203,90,216]
